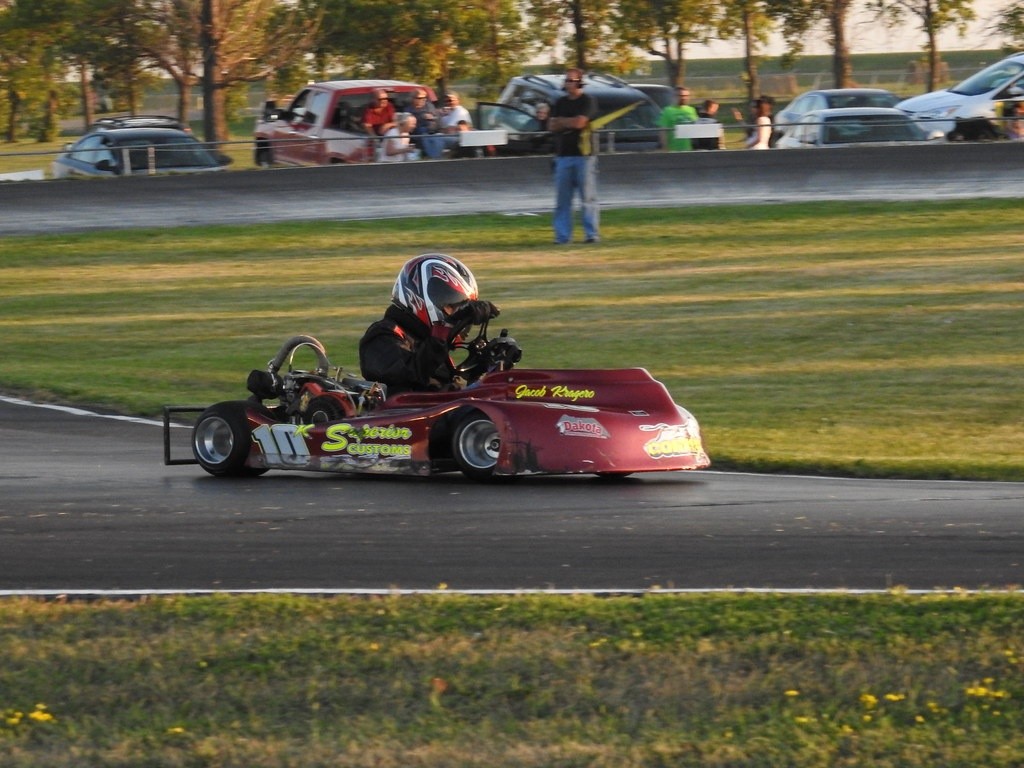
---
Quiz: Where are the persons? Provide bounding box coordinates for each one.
[548,69,601,245]
[653,86,726,151]
[360,89,484,163]
[360,253,503,392]
[994,87,1024,139]
[522,103,552,132]
[732,95,776,150]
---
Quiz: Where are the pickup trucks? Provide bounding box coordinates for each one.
[255,79,452,165]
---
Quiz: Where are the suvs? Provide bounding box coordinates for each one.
[628,84,677,109]
[476,71,665,154]
[84,115,192,134]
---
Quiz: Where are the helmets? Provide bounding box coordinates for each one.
[390,253,478,349]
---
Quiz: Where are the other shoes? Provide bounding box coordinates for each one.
[553,237,567,244]
[585,237,597,243]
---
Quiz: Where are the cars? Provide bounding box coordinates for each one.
[769,87,901,149]
[775,107,936,143]
[893,53,1024,141]
[53,128,234,175]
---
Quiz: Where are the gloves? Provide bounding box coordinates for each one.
[445,301,500,341]
[483,329,522,370]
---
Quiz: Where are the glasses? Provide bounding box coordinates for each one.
[565,79,580,82]
